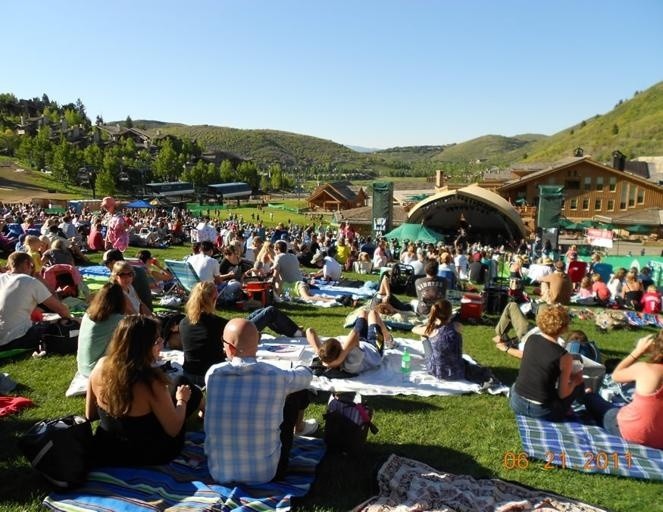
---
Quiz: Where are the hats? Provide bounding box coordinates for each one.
[99,248,122,265]
[310,253,323,264]
[569,253,577,258]
[100,197,115,208]
[555,260,564,270]
[544,257,553,264]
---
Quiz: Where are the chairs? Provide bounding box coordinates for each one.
[163,259,230,309]
[121,256,176,306]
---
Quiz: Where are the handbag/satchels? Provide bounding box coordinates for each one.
[323,398,378,449]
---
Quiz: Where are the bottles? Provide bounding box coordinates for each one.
[401,347,411,373]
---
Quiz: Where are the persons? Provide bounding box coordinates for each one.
[0,196,185,281]
[84,314,205,467]
[492,302,586,359]
[411,259,447,318]
[410,250,426,276]
[305,310,398,375]
[204,318,319,486]
[437,252,457,278]
[584,326,663,450]
[566,253,576,274]
[566,245,576,261]
[542,240,551,255]
[589,254,599,275]
[509,304,585,422]
[606,267,625,299]
[411,300,465,380]
[641,286,662,315]
[179,281,229,387]
[525,241,531,256]
[517,238,526,254]
[616,272,644,309]
[468,252,488,284]
[481,251,489,265]
[509,256,523,279]
[77,283,126,378]
[103,249,152,311]
[109,262,154,319]
[401,245,414,264]
[638,267,652,282]
[579,276,593,299]
[533,237,542,260]
[159,306,307,357]
[390,238,396,256]
[592,274,610,303]
[427,243,436,259]
[455,243,468,280]
[538,261,571,307]
[0,251,81,350]
[187,204,389,303]
[370,274,411,314]
[536,258,552,285]
[527,257,542,283]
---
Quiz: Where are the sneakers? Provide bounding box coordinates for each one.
[384,335,399,349]
[295,418,318,436]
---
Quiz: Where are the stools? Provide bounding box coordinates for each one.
[247,282,273,308]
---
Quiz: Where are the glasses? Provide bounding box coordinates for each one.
[221,336,231,345]
[225,252,232,256]
[117,272,133,277]
